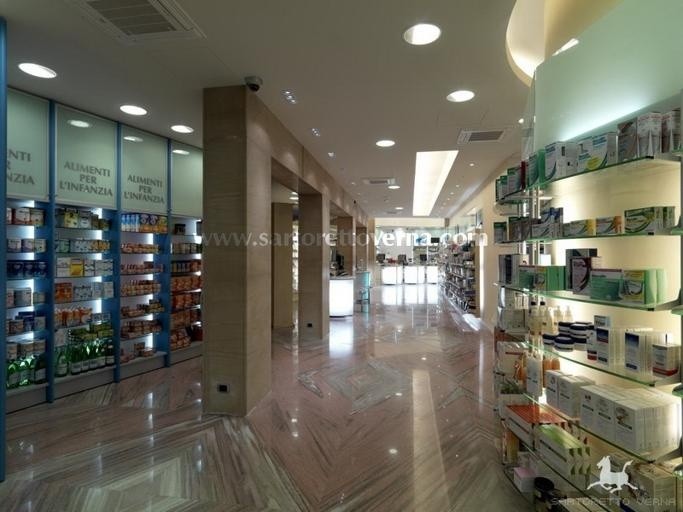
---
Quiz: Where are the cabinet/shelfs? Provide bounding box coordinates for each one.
[3,195,202,393]
[438,244,482,315]
[495,152,683,511]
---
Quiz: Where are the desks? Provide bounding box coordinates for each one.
[375,262,436,284]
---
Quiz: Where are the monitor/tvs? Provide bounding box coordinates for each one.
[332,250,345,271]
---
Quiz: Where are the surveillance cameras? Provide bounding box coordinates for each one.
[245,76,263,92]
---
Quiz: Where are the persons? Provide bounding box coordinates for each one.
[336,266,347,276]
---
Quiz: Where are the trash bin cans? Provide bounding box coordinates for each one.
[360,287,371,313]
